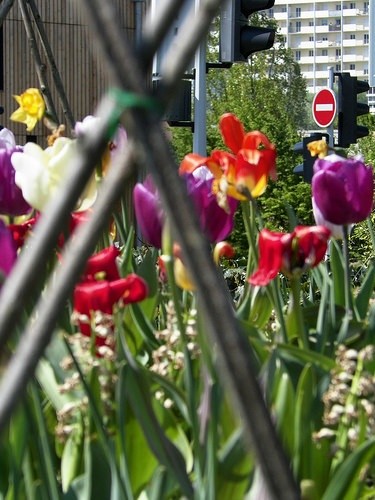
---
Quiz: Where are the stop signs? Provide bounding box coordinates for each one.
[310,89,335,129]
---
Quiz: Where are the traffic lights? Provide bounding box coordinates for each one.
[334,72,370,147]
[293,132,329,185]
[233,1,276,64]
